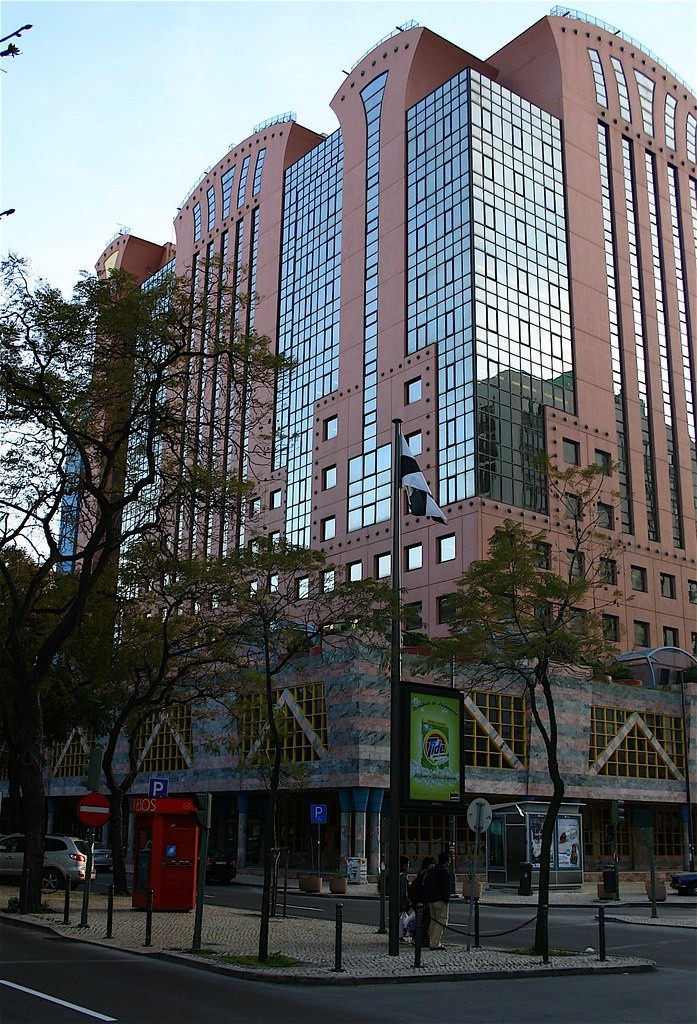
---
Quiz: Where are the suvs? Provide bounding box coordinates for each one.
[0,831,96,891]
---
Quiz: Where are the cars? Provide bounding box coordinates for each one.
[670,871,697,896]
[83,840,115,873]
[198,850,237,885]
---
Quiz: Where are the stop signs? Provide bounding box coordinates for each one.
[77,793,112,828]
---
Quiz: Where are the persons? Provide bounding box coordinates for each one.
[399,852,451,951]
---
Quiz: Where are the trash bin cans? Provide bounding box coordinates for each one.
[518,861,534,896]
[602,864,616,892]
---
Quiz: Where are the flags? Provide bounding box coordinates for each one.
[396,430,450,526]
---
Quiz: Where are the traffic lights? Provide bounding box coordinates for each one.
[639,826,656,849]
[612,800,626,824]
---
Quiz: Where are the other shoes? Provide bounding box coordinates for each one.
[430,944,446,951]
[419,939,430,948]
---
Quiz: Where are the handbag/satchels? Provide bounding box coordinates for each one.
[398,907,417,937]
[407,870,430,903]
